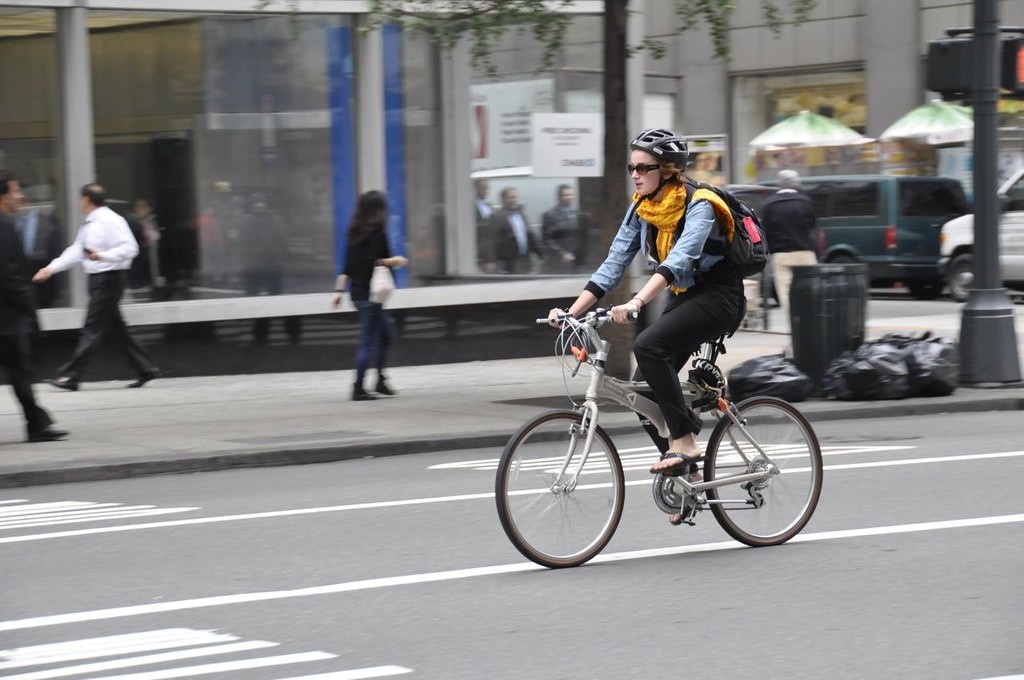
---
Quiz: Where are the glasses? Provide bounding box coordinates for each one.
[628,163,660,176]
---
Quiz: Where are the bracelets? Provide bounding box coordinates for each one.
[633,296,644,306]
[335,289,343,293]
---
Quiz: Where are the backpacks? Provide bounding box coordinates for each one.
[682,174,768,276]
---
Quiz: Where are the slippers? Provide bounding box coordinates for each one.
[650,452,708,473]
[670,478,707,525]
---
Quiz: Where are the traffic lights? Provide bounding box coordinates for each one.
[1000,37,1024,95]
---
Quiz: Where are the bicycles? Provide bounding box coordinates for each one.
[494,305,824,568]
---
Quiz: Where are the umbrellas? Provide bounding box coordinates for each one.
[748,108,878,153]
[876,96,974,145]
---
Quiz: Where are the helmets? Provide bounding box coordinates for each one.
[630,129,688,172]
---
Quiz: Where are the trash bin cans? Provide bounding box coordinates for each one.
[789,263,869,386]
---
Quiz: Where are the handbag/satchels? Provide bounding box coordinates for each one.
[369,258,396,304]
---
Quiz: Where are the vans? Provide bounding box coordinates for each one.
[935,169,1024,305]
[753,173,968,300]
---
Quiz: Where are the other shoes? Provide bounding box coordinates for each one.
[375,379,394,395]
[353,382,377,400]
[46,376,78,391]
[129,368,163,388]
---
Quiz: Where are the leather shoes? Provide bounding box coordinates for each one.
[29,422,68,441]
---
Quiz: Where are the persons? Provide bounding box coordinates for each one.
[760,168,816,336]
[540,184,590,273]
[118,197,165,304]
[548,129,746,526]
[0,167,70,440]
[471,178,496,268]
[241,191,289,298]
[332,190,408,400]
[31,181,162,392]
[486,185,544,273]
